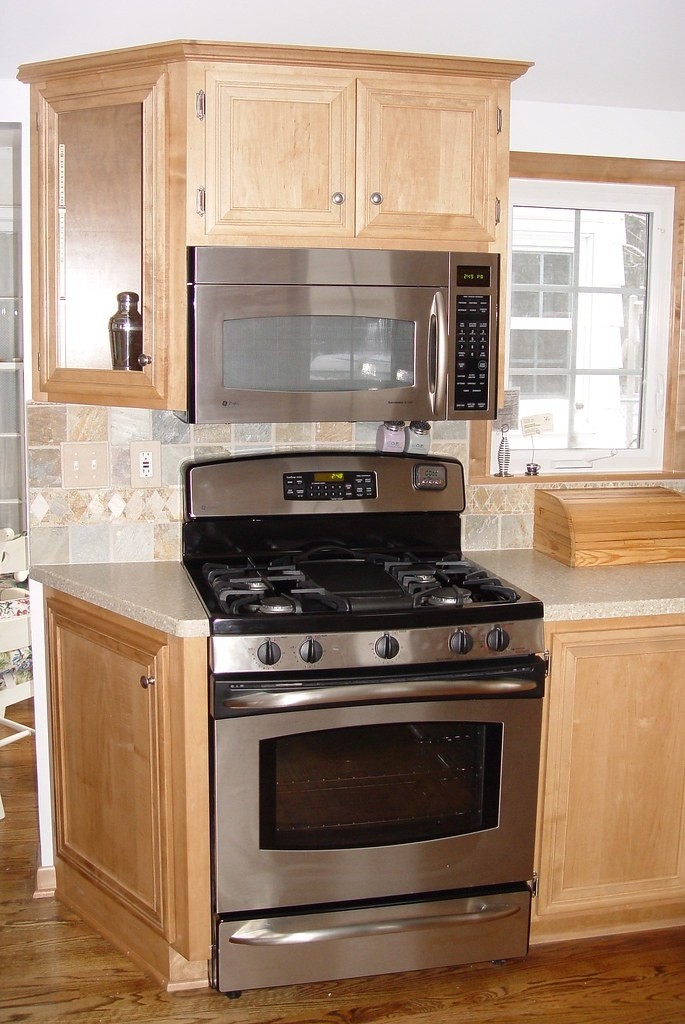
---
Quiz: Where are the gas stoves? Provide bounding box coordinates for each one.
[180,452,546,673]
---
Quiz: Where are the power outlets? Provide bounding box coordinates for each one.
[130,440,163,488]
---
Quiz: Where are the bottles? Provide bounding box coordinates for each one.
[405,421,431,454]
[375,421,405,454]
[108,291,142,370]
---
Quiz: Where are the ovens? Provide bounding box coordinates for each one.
[207,661,546,991]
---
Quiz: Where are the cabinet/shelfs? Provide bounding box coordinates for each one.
[528,384,685,946]
[39,585,214,991]
[187,58,512,245]
[31,59,186,411]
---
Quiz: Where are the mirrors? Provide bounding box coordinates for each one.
[55,101,141,373]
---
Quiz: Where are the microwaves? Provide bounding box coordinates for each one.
[172,246,499,424]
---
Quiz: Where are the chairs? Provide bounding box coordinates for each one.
[0,575,35,822]
